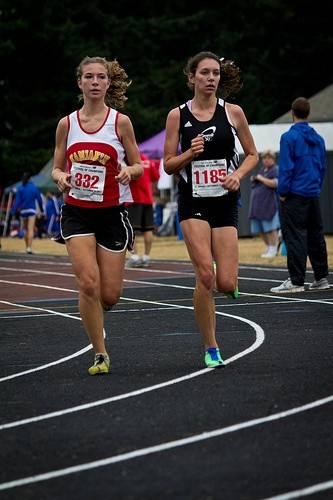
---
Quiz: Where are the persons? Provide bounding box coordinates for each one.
[270,96,330,293]
[163,51,259,368]
[248,149,280,258]
[6,189,64,240]
[125,152,160,269]
[52,56,144,376]
[12,171,44,253]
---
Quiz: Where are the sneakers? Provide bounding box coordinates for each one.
[269,278,305,293]
[308,278,330,290]
[213,261,238,301]
[88,353,111,375]
[204,347,226,368]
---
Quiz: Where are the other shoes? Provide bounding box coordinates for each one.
[141,255,150,266]
[27,248,32,254]
[130,255,141,266]
[261,245,277,258]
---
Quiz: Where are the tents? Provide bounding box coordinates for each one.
[1,157,61,237]
[136,121,333,238]
